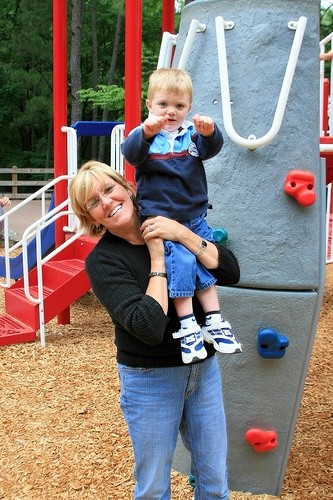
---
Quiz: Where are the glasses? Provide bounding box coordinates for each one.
[86,180,115,210]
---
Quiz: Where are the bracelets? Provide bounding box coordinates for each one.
[194,237,208,258]
[148,272,167,279]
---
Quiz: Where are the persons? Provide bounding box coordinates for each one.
[0,197,11,208]
[120,66,244,365]
[68,161,242,499]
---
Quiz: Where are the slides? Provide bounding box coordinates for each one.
[0,192,55,280]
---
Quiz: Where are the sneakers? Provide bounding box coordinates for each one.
[171,324,206,364]
[201,318,242,354]
